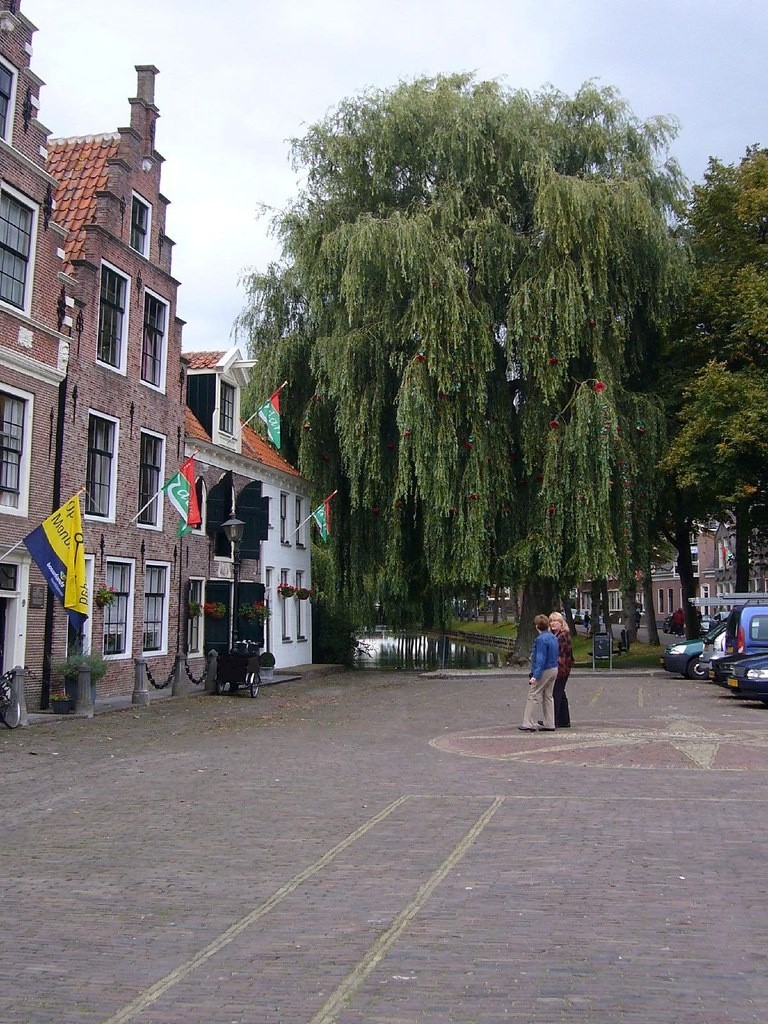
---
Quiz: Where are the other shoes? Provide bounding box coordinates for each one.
[538,720,543,725]
[518,726,537,732]
[555,723,570,728]
[538,727,555,731]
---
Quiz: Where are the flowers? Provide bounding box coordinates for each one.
[97,584,117,606]
[277,583,298,599]
[296,587,315,602]
[49,690,74,701]
[205,602,226,614]
[237,601,272,626]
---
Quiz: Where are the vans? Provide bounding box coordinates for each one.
[722,602,768,655]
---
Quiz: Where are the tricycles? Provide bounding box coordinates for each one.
[211,640,265,698]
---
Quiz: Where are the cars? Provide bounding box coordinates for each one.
[683,614,712,634]
[711,653,768,707]
[561,607,603,626]
[662,613,673,634]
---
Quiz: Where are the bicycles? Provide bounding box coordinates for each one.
[0,670,22,729]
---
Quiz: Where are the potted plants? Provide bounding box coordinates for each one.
[188,602,203,619]
[54,644,109,710]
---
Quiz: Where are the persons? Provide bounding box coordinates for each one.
[518,614,559,732]
[584,612,590,634]
[636,611,641,628]
[673,608,686,637]
[228,649,239,693]
[548,612,574,727]
[696,607,702,622]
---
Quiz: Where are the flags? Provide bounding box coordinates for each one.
[164,455,201,538]
[23,495,89,628]
[257,386,282,449]
[313,493,335,543]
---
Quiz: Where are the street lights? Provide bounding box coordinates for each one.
[220,513,247,655]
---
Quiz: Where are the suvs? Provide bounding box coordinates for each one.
[698,612,759,683]
[659,621,727,680]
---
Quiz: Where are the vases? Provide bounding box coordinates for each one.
[51,701,72,714]
